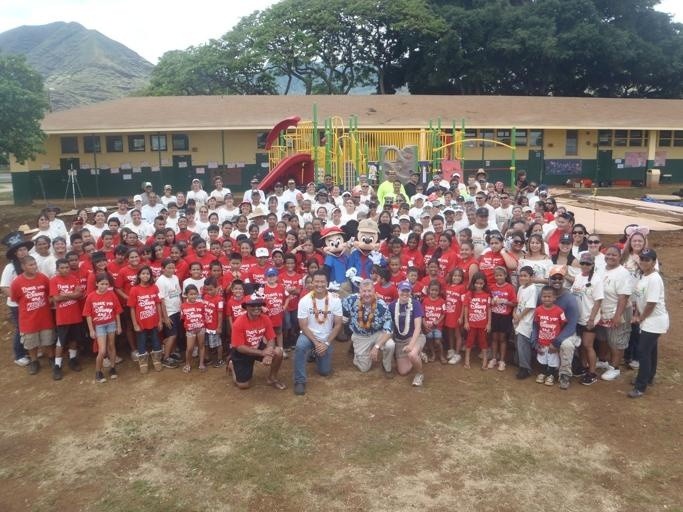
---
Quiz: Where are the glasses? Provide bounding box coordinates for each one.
[573,231,584,234]
[588,240,599,244]
[581,262,592,265]
[551,276,562,280]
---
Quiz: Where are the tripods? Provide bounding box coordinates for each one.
[61,170,85,207]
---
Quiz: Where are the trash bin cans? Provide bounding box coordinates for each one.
[646,169,661,187]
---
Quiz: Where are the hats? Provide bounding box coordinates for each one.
[397,281,411,291]
[579,253,594,264]
[241,293,264,307]
[266,268,278,276]
[639,248,656,258]
[559,234,573,243]
[549,266,564,277]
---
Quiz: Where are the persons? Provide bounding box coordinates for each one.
[2,169,671,400]
